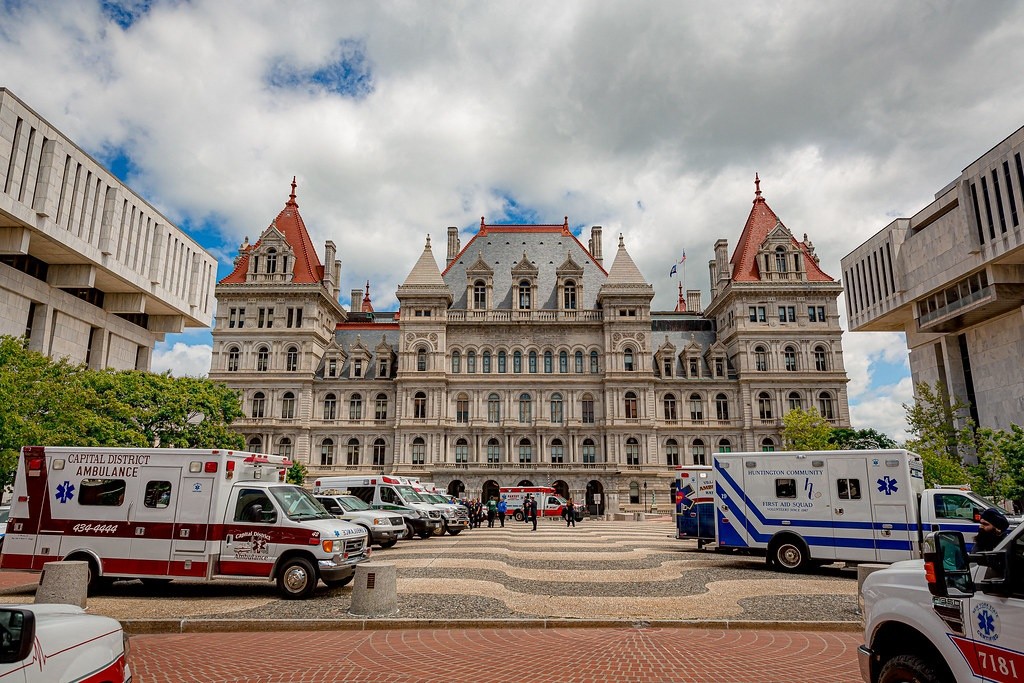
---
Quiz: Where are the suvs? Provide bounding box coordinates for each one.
[287,496,408,549]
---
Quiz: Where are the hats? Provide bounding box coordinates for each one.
[531,495,535,497]
[980,508,1010,532]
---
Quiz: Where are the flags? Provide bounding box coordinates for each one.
[678,251,686,264]
[670,265,676,277]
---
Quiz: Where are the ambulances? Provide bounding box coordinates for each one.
[675,464,715,545]
[712,449,1024,574]
[856,523,1024,683]
[1,604,131,683]
[500,486,590,522]
[0,444,371,600]
[313,473,470,540]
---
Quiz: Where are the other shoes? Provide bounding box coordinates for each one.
[531,529,536,531]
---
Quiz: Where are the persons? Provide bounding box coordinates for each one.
[520,496,537,531]
[424,486,441,494]
[498,498,507,528]
[566,498,575,528]
[414,481,422,492]
[486,496,496,527]
[460,497,482,528]
[971,508,1016,579]
[449,497,457,504]
